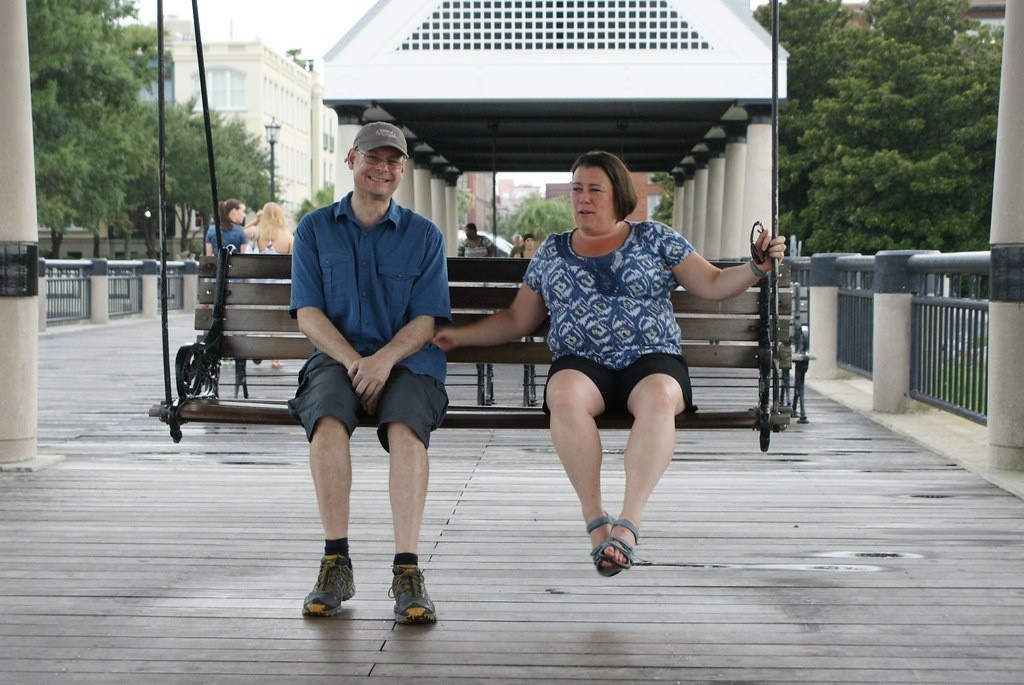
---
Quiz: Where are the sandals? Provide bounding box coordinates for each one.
[586,510,623,577]
[598,518,640,569]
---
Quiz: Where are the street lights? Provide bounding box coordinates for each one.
[265,113,283,205]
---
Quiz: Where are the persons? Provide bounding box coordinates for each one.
[205,121,787,624]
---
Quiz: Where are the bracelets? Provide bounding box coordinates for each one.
[750,260,767,279]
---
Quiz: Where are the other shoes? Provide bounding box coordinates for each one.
[222,357,234,365]
[272,360,283,367]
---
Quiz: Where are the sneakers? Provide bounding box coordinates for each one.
[302,553,356,616]
[387,565,437,625]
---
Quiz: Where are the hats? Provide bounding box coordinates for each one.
[353,122,410,159]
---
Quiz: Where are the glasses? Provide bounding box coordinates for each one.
[357,149,406,170]
